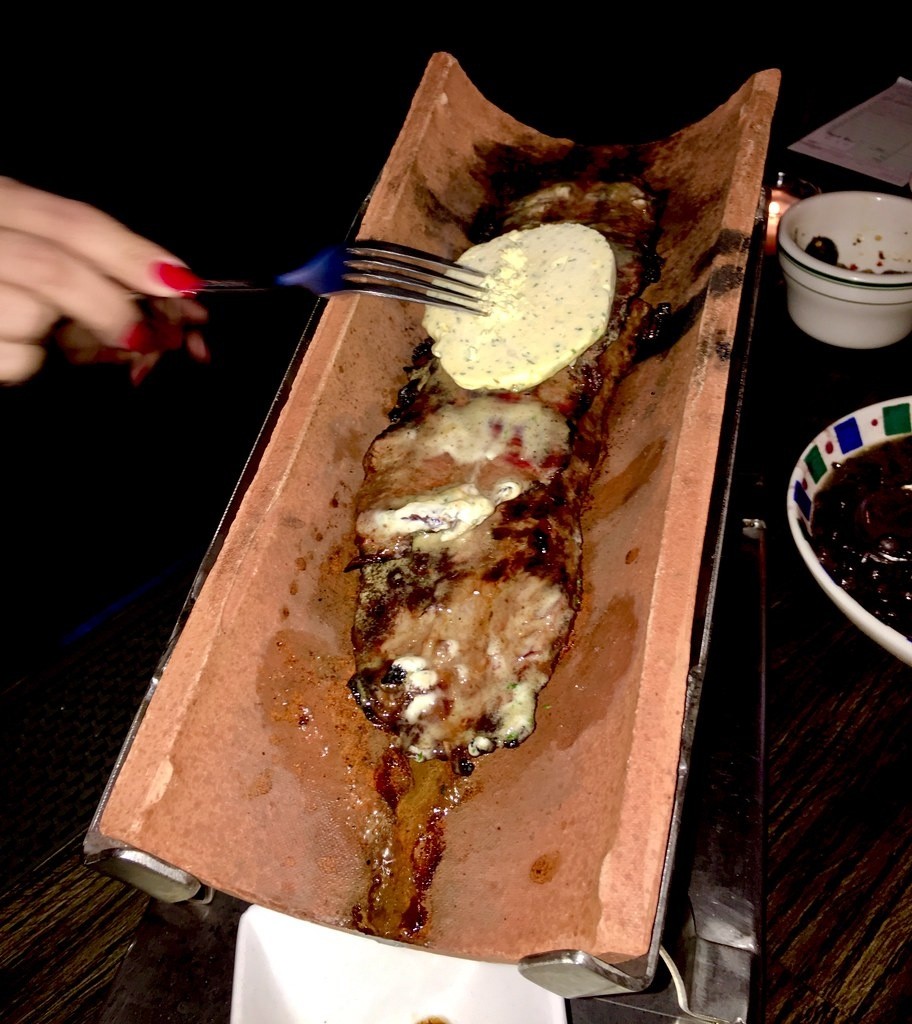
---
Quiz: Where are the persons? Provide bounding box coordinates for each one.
[2,177,200,384]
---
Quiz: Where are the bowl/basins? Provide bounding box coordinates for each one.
[786,394,911,668]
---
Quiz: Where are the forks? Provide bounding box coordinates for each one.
[129,241,491,320]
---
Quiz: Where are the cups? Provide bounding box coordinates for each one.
[764,168,823,256]
[778,187,912,350]
